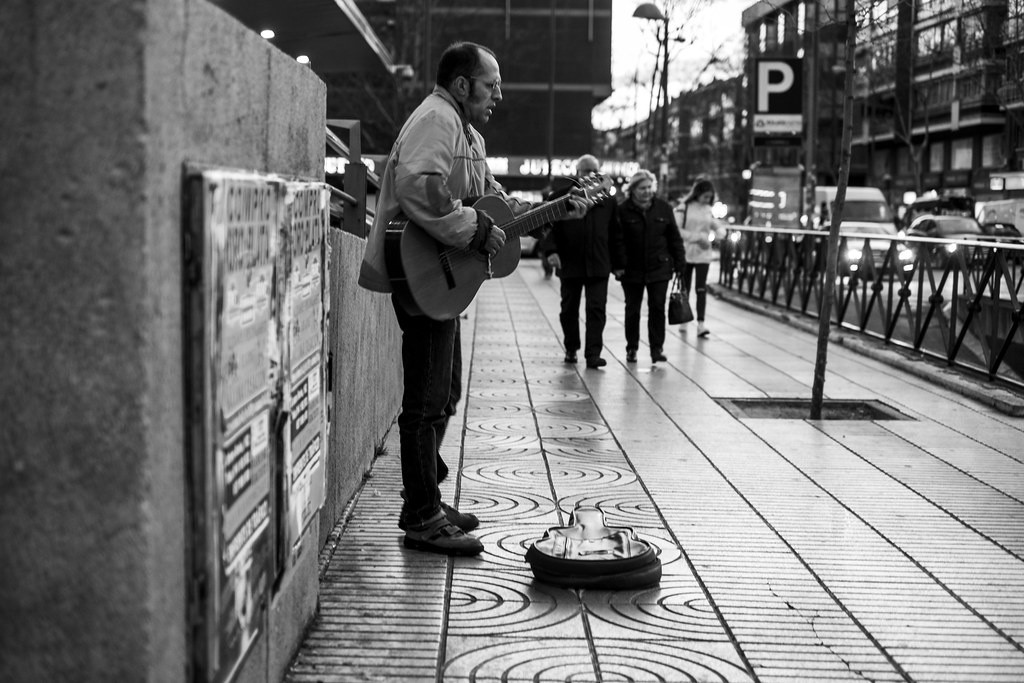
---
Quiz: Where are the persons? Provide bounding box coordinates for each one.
[358,42,594,557]
[539,154,686,368]
[675,180,721,337]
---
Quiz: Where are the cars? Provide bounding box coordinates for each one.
[903,215,992,267]
[982,220,1024,260]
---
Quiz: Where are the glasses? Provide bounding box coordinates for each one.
[467,75,500,89]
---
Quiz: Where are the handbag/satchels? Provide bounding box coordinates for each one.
[669,272,694,325]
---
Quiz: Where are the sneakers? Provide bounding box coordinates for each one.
[436,501,480,532]
[403,516,483,556]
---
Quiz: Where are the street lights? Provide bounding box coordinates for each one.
[631,3,669,203]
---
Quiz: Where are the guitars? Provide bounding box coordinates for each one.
[384,170,615,327]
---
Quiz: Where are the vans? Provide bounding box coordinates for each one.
[813,183,916,281]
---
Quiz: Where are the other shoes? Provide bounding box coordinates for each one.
[678,323,688,332]
[587,356,606,367]
[697,324,709,337]
[652,352,667,363]
[565,348,579,363]
[627,350,637,362]
